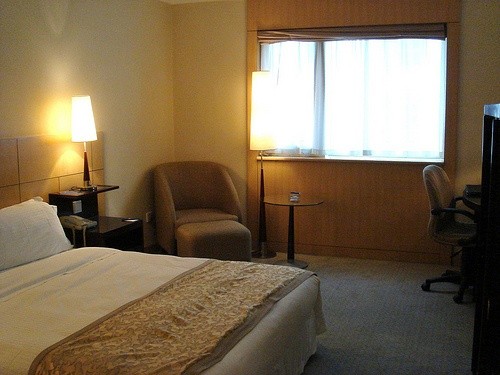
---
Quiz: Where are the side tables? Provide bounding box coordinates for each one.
[262,193,325,269]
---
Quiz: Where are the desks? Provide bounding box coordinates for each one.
[462,185,486,282]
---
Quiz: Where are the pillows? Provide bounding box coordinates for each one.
[0,196,74,270]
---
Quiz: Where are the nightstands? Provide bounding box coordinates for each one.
[51,184,144,252]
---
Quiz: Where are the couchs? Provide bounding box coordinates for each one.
[151,161,252,261]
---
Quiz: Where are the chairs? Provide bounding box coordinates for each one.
[421,164,480,303]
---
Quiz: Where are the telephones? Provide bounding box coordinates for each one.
[59,215,97,230]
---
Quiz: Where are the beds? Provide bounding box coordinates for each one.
[0,249,327,375]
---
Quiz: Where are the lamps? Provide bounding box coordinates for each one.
[249,71,281,259]
[71,94,100,185]
